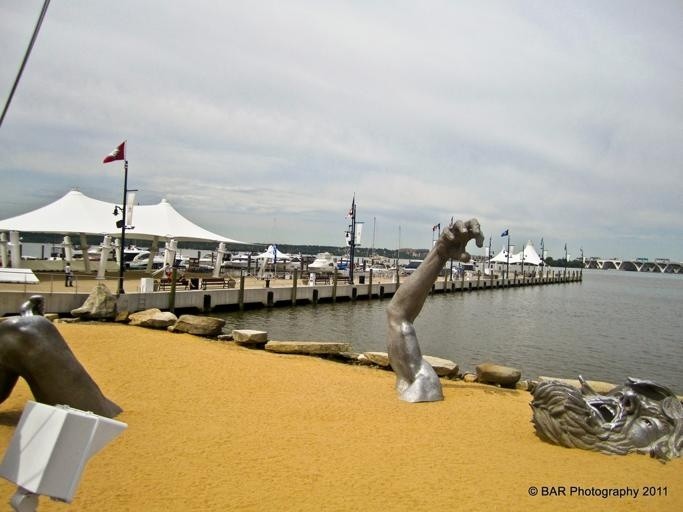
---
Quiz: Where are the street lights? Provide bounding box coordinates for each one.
[487,235,583,278]
[112,158,134,293]
[345,201,356,283]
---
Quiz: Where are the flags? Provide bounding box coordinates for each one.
[501,230,509,237]
[102,140,124,163]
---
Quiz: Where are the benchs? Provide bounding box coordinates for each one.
[306,275,352,286]
[154,274,234,291]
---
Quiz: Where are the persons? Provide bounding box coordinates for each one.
[0,314,123,419]
[383,214,486,403]
[64,262,72,287]
[526,370,683,463]
